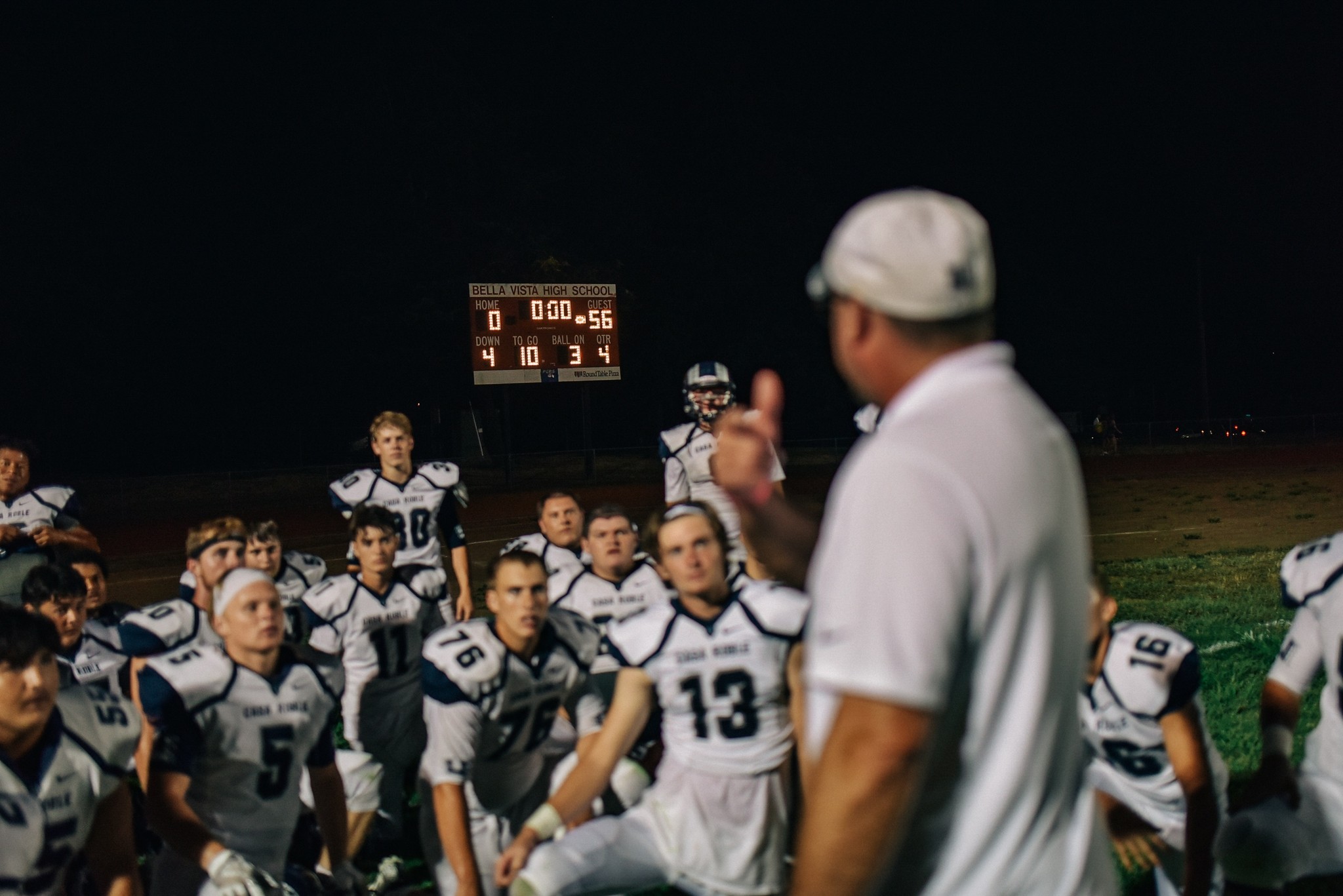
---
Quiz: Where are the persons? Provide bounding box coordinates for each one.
[0,363,810,894]
[790,189,1122,896]
[1078,573,1230,895]
[1216,533,1343,896]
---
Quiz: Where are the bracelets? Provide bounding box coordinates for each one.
[1263,726,1293,759]
[525,802,565,841]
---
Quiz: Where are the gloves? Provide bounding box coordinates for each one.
[206,852,278,896]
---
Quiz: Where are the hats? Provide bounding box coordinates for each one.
[805,186,996,323]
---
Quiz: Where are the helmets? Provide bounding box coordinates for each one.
[683,359,736,419]
